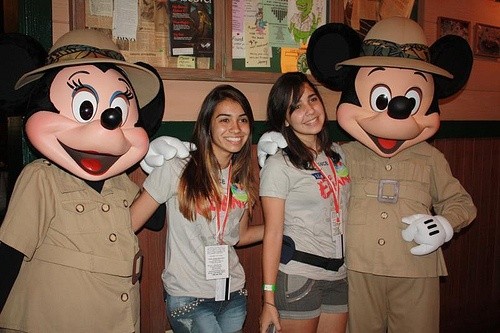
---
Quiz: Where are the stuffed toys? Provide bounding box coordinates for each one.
[0,30,198,333]
[257,17,477,333]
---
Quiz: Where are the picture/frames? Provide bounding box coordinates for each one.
[473,20,500,60]
[436,15,473,59]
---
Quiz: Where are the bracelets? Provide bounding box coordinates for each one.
[264,302,277,308]
[262,284,276,291]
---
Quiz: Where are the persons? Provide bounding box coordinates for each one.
[259,71,350,333]
[130,84,266,333]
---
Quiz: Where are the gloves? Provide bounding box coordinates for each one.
[139,135,196,176]
[257,131,288,169]
[401,214,453,257]
